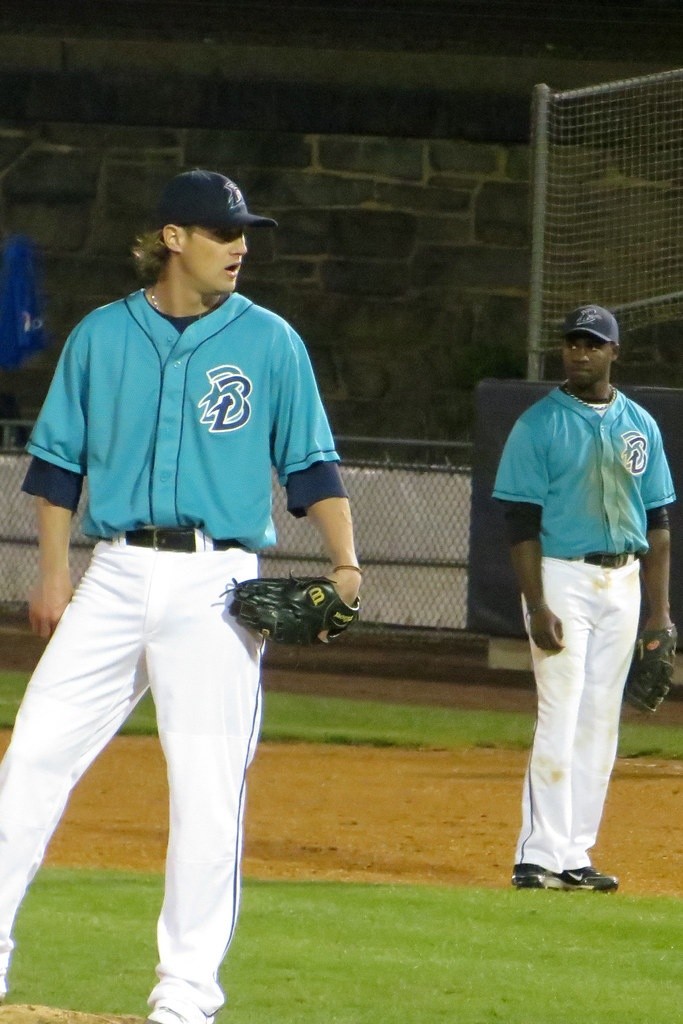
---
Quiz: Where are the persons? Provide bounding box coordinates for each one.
[0,170,362,1024]
[491,304,678,892]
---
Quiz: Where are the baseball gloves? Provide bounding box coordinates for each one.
[622,624,677,718]
[226,576,359,646]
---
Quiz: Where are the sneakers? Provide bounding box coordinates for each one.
[511,862,548,888]
[546,866,618,892]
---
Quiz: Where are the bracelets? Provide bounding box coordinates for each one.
[528,603,547,613]
[333,565,362,574]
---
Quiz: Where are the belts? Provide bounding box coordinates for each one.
[98,528,244,551]
[568,552,640,569]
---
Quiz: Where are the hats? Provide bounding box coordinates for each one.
[158,167,279,228]
[560,304,619,345]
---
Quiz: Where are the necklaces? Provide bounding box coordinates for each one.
[152,286,158,309]
[564,379,617,409]
